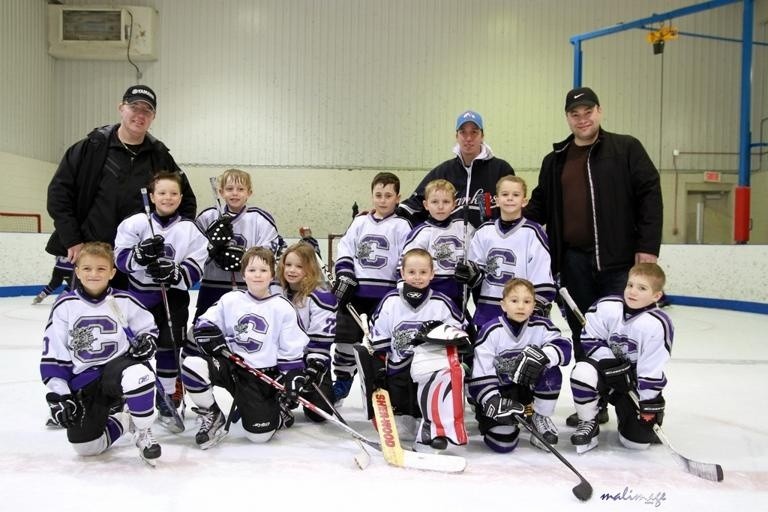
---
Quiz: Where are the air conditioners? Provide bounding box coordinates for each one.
[46,3,159,64]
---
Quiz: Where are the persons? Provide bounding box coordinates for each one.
[33,86,673,464]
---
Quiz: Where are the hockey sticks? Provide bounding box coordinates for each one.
[310,382,371,472]
[558,287,722,483]
[222,350,415,454]
[362,314,466,472]
[461,363,593,501]
[104,296,184,434]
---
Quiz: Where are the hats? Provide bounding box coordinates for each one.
[123,85,157,111]
[458,110,484,129]
[565,87,600,112]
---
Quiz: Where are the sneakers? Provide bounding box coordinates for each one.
[156,375,187,416]
[566,409,610,427]
[192,403,224,444]
[425,438,449,449]
[570,417,600,445]
[530,409,558,444]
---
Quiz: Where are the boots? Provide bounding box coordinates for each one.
[332,368,358,404]
[127,414,161,459]
[281,405,293,428]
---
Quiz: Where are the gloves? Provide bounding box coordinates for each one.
[640,396,664,430]
[193,326,227,358]
[206,219,246,271]
[281,354,331,409]
[129,335,158,361]
[133,236,182,286]
[484,394,524,426]
[598,360,636,405]
[532,299,554,318]
[46,394,83,429]
[452,258,484,290]
[331,272,358,314]
[509,345,549,390]
